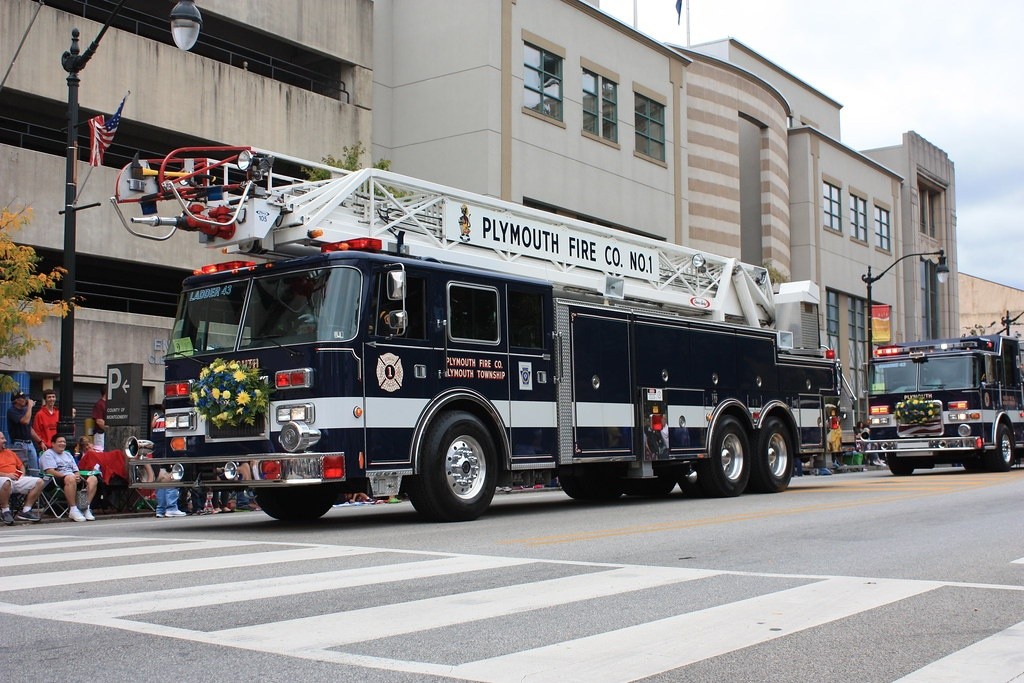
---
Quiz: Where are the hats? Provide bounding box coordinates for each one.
[11,390,30,400]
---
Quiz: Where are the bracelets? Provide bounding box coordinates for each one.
[38,439,43,444]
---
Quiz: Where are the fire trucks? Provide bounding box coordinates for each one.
[856,331,1024,473]
[111,146,843,524]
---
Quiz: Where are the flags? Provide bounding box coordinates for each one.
[88,91,129,168]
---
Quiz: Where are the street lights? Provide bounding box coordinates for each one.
[55,0,204,459]
[861,248,950,360]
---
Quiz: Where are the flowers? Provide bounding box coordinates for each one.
[189,358,276,428]
[893,395,940,426]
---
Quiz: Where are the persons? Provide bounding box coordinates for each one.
[335,490,409,505]
[860,427,886,467]
[6,389,47,512]
[644,413,668,461]
[38,433,98,521]
[791,454,803,477]
[853,422,868,453]
[33,389,60,455]
[298,287,401,338]
[183,488,264,515]
[73,407,77,441]
[92,384,107,451]
[151,398,186,518]
[826,422,843,468]
[0,432,44,523]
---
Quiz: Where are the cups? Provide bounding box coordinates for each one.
[26,401,35,407]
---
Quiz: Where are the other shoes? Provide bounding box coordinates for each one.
[179,493,403,517]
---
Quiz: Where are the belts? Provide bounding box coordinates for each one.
[14,439,32,444]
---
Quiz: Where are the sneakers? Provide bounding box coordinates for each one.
[0,509,14,524]
[165,508,186,517]
[83,504,95,520]
[156,513,166,517]
[17,509,40,521]
[69,509,86,521]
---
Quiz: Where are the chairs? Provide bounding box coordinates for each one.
[13,447,157,520]
